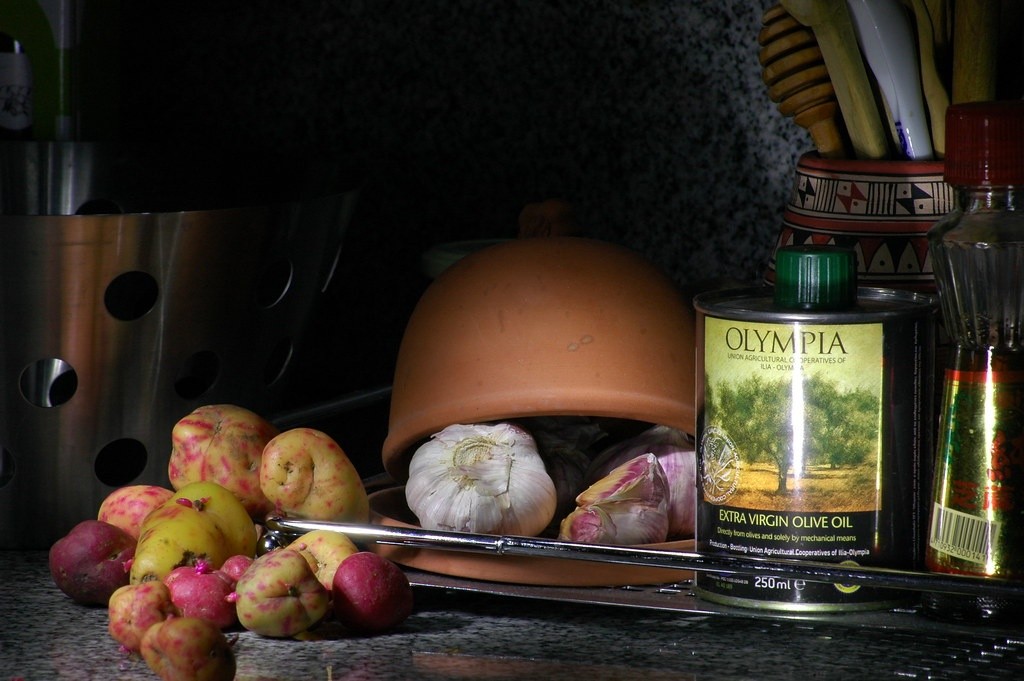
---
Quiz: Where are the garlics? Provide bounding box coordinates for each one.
[404,415,697,546]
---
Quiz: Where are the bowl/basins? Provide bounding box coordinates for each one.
[381,237,702,483]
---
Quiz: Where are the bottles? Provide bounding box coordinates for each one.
[924,100,1024,622]
[690,245,942,614]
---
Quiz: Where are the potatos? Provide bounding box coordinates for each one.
[48,404,412,681]
